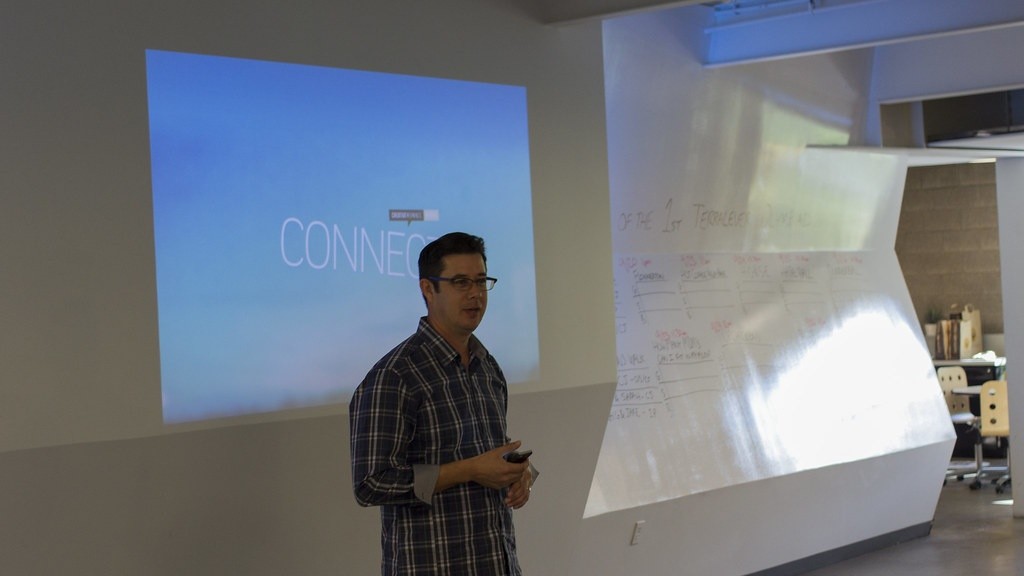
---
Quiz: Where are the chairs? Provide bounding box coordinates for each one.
[965,379,1012,494]
[937,366,992,486]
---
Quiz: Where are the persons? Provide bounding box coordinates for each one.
[348,231,541,575]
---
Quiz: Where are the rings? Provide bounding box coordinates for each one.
[527,488,531,493]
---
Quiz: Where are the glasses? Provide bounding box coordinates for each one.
[427,277,497,292]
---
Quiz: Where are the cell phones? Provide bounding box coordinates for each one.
[507,450,533,463]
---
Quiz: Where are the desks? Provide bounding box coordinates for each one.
[951,384,999,481]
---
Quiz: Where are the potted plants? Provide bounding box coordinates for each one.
[923,306,944,338]
